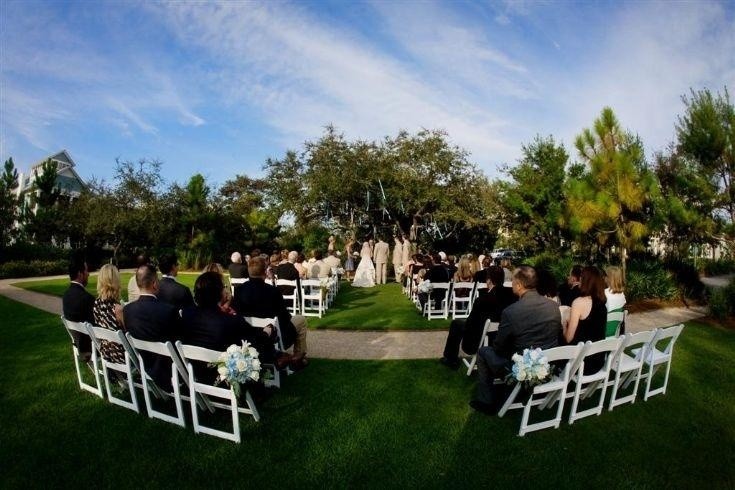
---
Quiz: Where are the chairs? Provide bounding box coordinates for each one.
[84,322,160,417]
[398,267,525,376]
[599,326,657,413]
[125,332,207,431]
[499,342,583,437]
[633,325,682,400]
[569,329,621,420]
[175,336,261,443]
[223,272,342,386]
[58,314,127,400]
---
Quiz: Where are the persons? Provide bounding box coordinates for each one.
[442,266,626,414]
[329,236,410,287]
[403,250,516,315]
[64,254,306,408]
[231,248,341,314]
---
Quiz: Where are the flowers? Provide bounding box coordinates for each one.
[215,337,261,383]
[513,345,551,389]
